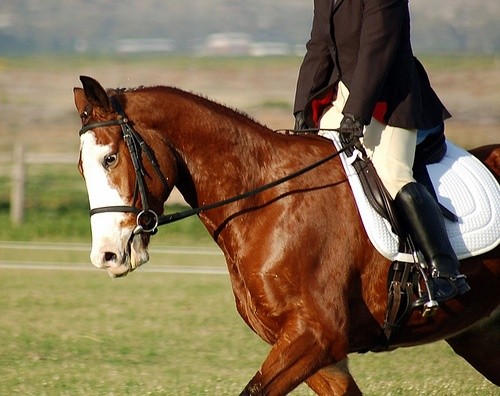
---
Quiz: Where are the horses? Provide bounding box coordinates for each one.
[73,75,500,395]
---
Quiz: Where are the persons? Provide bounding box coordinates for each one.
[292,0,471,309]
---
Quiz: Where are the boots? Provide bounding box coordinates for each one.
[395,182,471,310]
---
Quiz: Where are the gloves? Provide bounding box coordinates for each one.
[294,112,309,129]
[339,115,363,157]
